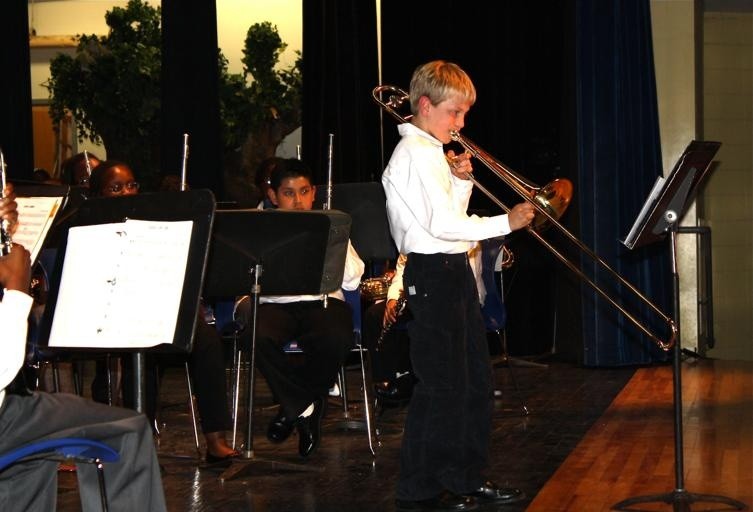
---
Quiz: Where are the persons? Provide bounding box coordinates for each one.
[60,153,103,182]
[383,60,538,507]
[253,157,285,209]
[378,239,487,408]
[234,157,365,455]
[1,182,167,510]
[86,162,245,464]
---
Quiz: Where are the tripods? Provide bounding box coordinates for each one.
[204,267,327,483]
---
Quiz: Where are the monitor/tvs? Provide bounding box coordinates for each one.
[612,230,753,512]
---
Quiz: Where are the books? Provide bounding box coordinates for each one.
[8,193,64,266]
[45,216,196,351]
[622,175,666,249]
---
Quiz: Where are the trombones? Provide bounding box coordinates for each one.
[372,84,679,353]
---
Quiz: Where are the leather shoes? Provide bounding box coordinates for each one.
[376,383,408,407]
[267,414,295,444]
[474,479,525,507]
[451,496,477,510]
[205,438,239,461]
[299,396,325,459]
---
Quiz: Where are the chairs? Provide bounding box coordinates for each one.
[205,283,381,460]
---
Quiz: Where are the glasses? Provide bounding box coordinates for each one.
[108,181,140,193]
